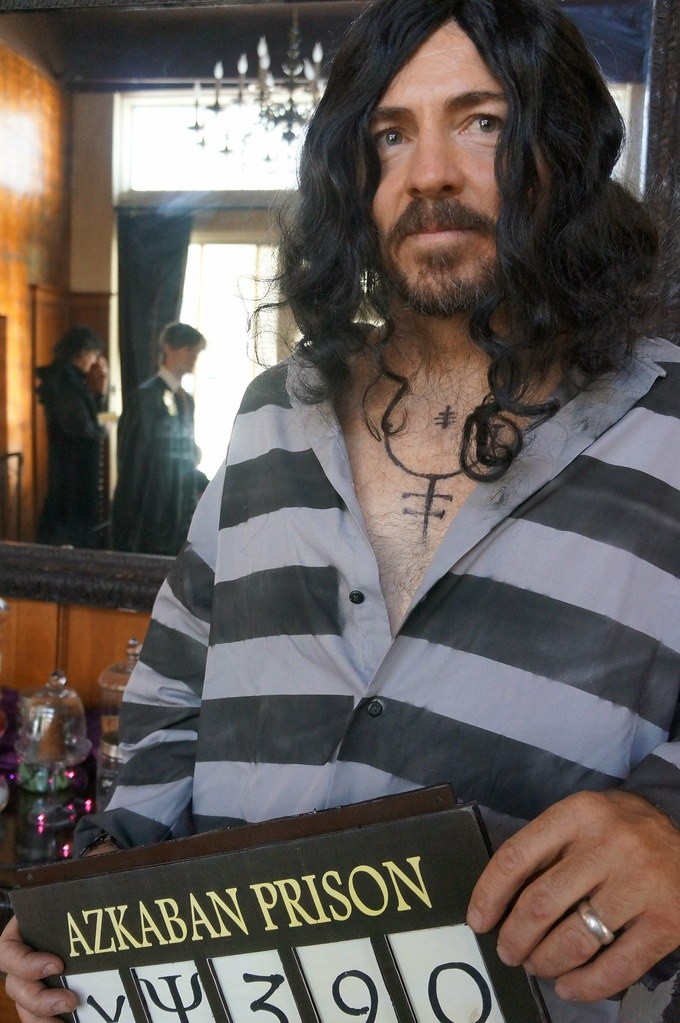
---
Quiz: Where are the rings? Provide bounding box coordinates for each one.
[577,900,615,946]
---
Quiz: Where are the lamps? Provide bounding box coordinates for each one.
[188,0,323,166]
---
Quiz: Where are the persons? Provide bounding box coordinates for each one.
[193,447,210,501]
[0,2,679,1022]
[34,325,109,546]
[111,322,211,557]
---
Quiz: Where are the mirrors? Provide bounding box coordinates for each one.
[0,0,653,558]
